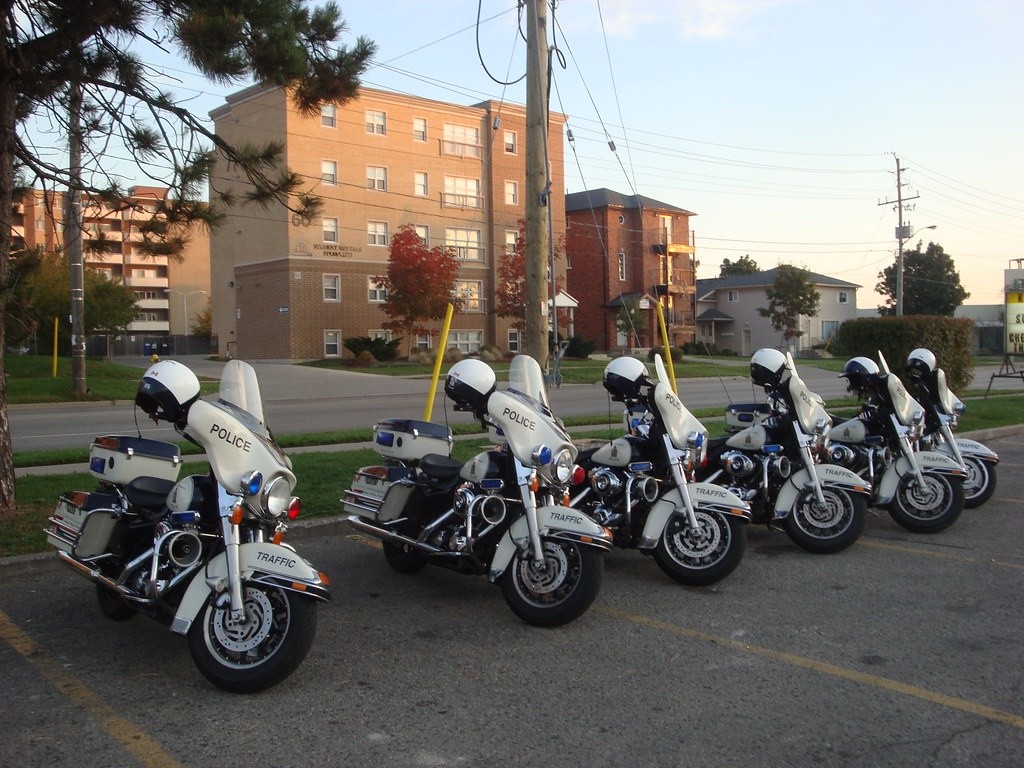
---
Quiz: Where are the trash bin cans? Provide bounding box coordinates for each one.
[144,337,169,356]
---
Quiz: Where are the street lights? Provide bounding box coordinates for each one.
[896,225,938,316]
[163,289,206,355]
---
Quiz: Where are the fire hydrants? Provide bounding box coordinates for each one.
[149,354,161,366]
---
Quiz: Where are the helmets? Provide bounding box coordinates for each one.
[603,356,649,398]
[444,359,496,411]
[135,360,200,424]
[837,356,880,393]
[907,348,936,377]
[750,348,788,385]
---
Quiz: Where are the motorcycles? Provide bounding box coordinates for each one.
[339,354,613,627]
[42,359,332,693]
[488,353,753,586]
[695,348,1000,554]
